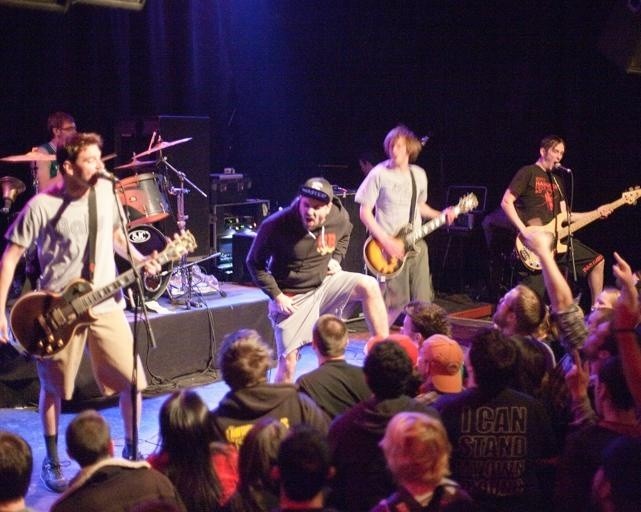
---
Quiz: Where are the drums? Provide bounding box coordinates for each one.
[114,224,173,302]
[116,172,170,227]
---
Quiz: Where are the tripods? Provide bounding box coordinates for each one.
[168,183,227,310]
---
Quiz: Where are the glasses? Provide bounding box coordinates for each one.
[59,126,78,134]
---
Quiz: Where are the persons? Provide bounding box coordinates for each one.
[353,123,460,357]
[500,133,615,307]
[245,176,391,385]
[1,326,324,512]
[296,251,640,512]
[1,129,164,495]
[356,147,383,176]
[34,109,77,194]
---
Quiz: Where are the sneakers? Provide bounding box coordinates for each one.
[120,446,147,463]
[41,458,70,492]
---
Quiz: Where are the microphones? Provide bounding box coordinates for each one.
[554,162,571,174]
[95,167,120,186]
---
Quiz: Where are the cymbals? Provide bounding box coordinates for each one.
[113,159,157,170]
[131,138,192,159]
[1,153,57,162]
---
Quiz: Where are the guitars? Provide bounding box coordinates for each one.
[363,192,479,280]
[9,228,198,360]
[515,185,641,273]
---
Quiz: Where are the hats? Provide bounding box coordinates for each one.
[424,333,464,394]
[301,178,334,204]
